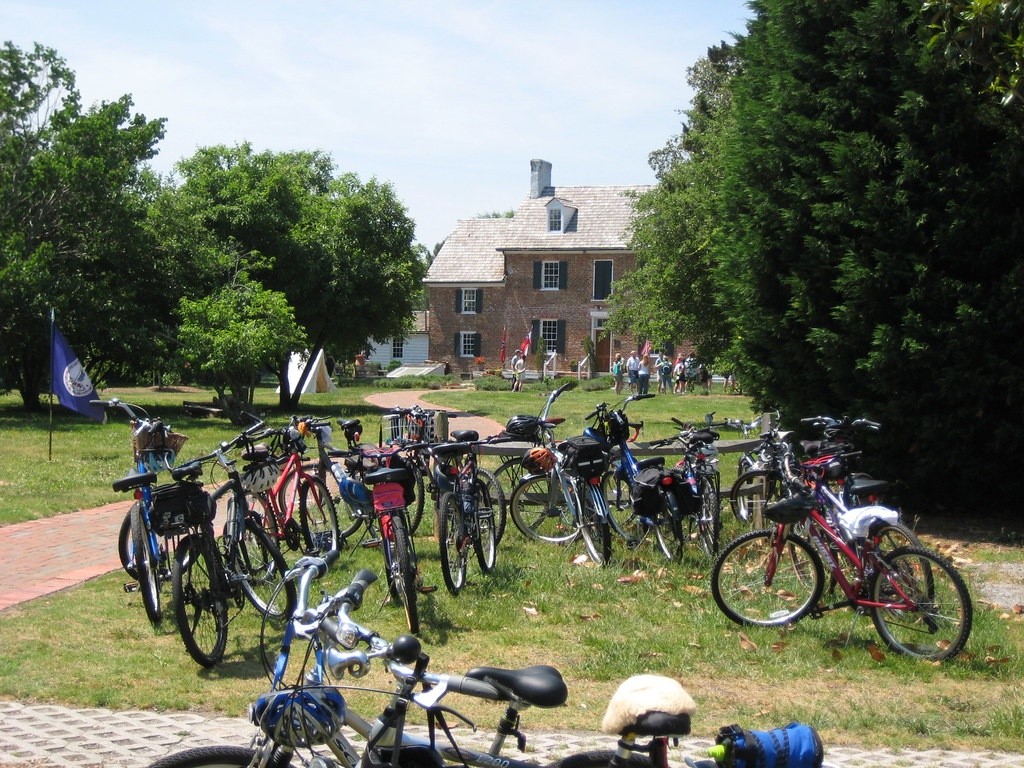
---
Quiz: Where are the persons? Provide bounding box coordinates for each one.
[626,351,640,394]
[637,354,650,395]
[610,352,625,395]
[723,373,737,393]
[655,351,712,394]
[511,350,526,392]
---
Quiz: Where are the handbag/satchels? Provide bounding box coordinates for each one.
[629,466,702,517]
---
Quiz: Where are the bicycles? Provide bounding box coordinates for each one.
[505,381,976,663]
[90,394,511,668]
[144,539,825,768]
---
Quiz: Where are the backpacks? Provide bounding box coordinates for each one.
[662,361,671,374]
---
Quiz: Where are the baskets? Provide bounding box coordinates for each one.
[390,406,437,443]
[130,420,188,462]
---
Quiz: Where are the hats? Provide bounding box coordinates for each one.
[515,350,523,354]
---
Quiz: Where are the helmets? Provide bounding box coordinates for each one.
[244,459,279,493]
[763,493,818,524]
[506,414,538,435]
[701,443,719,463]
[139,447,174,472]
[583,426,607,448]
[435,464,460,491]
[341,476,375,511]
[248,685,346,746]
[824,421,842,438]
[521,447,559,474]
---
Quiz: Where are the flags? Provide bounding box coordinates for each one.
[500,330,506,361]
[51,323,107,426]
[641,340,653,355]
[520,331,531,356]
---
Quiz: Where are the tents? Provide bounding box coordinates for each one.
[275,348,336,393]
